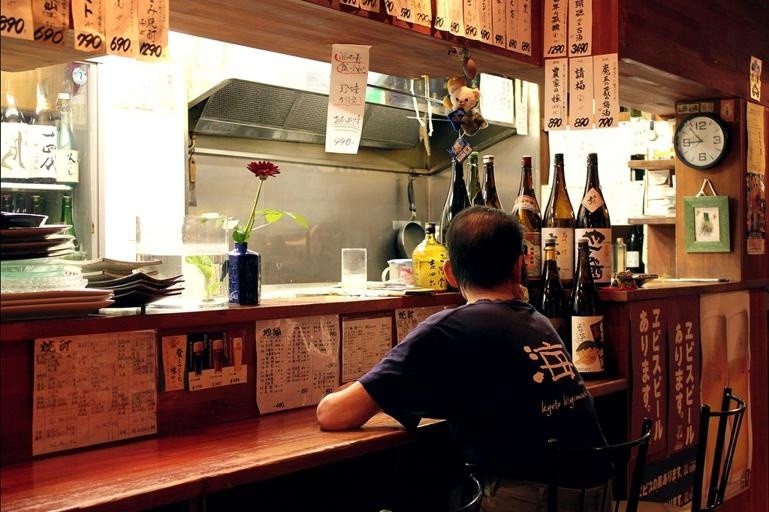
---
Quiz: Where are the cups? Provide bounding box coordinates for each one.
[339,247,368,297]
[193,337,244,377]
[382,258,414,289]
[182,214,229,307]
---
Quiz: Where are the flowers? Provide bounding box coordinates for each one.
[221,154,311,249]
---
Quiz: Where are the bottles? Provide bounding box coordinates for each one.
[57,92,80,186]
[466,151,484,207]
[540,237,571,359]
[627,225,644,273]
[508,153,611,289]
[481,154,504,211]
[0,68,30,184]
[30,67,58,185]
[0,192,49,226]
[439,155,473,247]
[412,221,449,289]
[568,239,609,380]
[612,237,629,279]
[60,195,80,253]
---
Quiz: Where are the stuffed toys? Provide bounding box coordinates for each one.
[448,84,489,137]
[442,76,466,131]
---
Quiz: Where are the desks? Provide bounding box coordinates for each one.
[0,377,631,512]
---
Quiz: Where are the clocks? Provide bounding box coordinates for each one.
[672,113,730,171]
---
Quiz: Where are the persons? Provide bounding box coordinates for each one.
[315,203,617,512]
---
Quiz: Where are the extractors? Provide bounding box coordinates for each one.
[184,75,517,177]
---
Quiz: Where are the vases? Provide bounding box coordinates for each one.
[223,243,259,304]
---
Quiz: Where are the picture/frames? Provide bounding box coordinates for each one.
[679,193,734,258]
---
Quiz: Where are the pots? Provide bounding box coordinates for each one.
[393,169,424,259]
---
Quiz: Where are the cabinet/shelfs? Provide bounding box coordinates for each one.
[627,156,677,281]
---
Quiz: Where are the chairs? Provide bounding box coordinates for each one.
[379,456,488,512]
[611,381,749,512]
[543,415,655,512]
